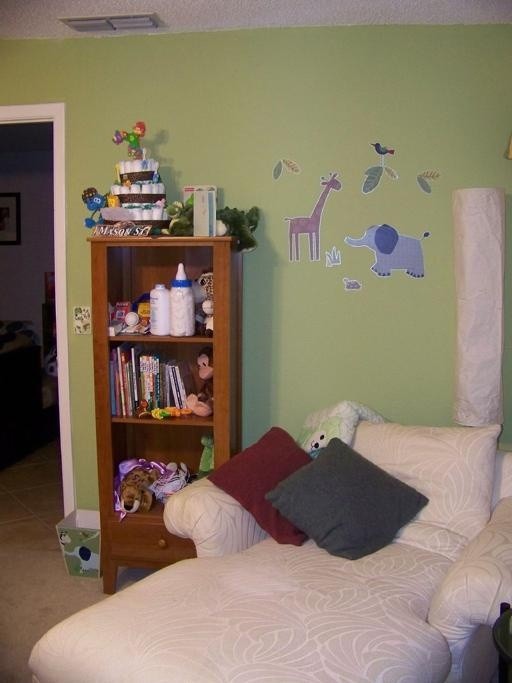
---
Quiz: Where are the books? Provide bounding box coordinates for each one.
[109,340,189,415]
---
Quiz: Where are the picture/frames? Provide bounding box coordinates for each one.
[0,192,21,246]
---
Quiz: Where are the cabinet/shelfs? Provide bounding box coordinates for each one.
[84,236,244,595]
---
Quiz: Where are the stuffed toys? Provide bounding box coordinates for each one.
[134,398,150,418]
[195,268,214,338]
[161,198,193,235]
[197,430,215,477]
[185,344,214,417]
[118,464,161,514]
[81,186,113,225]
[151,404,192,420]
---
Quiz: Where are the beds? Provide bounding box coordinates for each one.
[29,410,510,683]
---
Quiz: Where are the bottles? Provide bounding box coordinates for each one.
[148,262,197,337]
